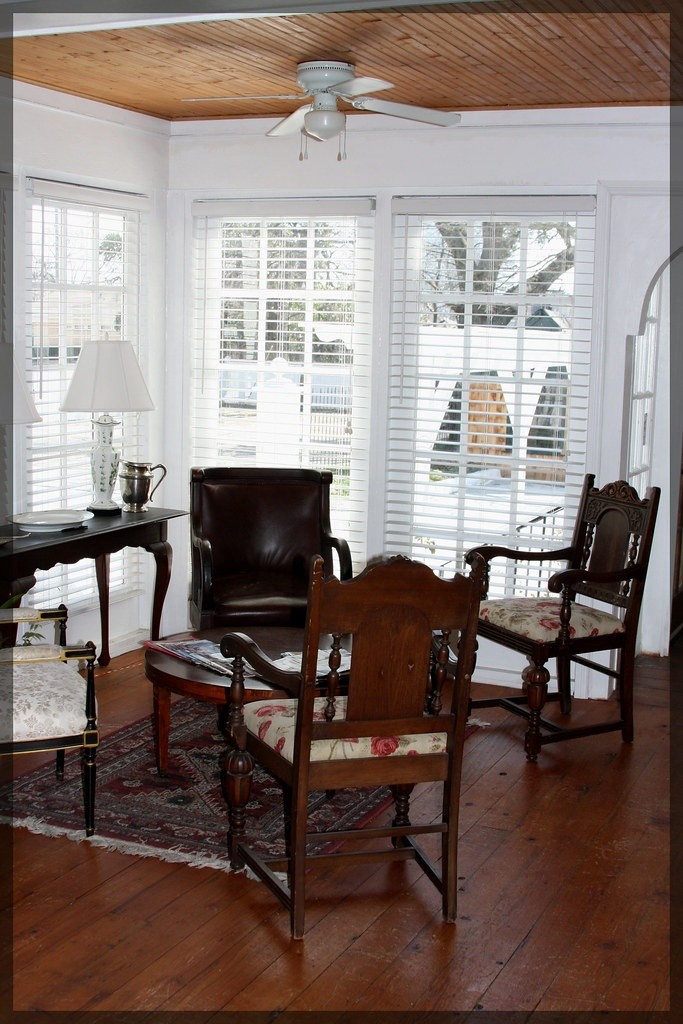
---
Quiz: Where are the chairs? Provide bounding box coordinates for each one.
[188,466,353,639]
[0,604,100,837]
[219,552,487,941]
[464,473,661,765]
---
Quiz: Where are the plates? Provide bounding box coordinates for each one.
[6,510,94,532]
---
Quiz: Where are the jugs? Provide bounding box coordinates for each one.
[118,461,167,513]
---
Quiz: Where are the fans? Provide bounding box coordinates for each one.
[180,61,461,159]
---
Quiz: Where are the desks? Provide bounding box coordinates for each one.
[0,507,191,668]
[145,629,352,780]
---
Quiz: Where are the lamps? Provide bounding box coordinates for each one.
[59,332,156,517]
[0,332,43,541]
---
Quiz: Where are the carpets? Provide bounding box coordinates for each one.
[0,694,493,888]
